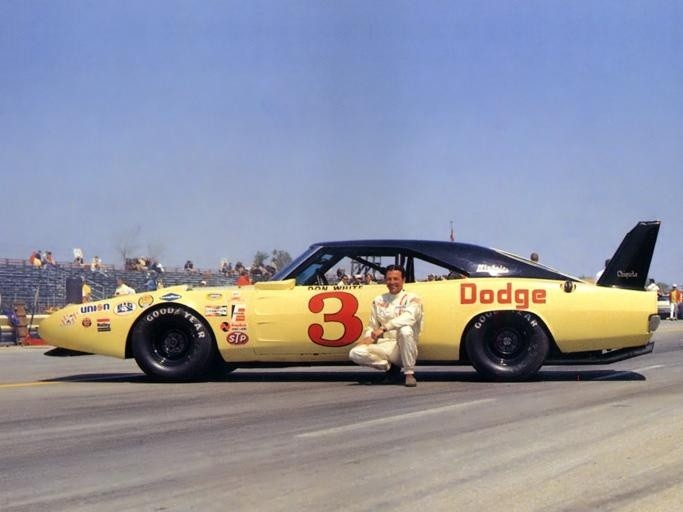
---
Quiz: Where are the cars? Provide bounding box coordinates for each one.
[36,219,659,383]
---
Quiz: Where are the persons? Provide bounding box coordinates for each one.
[115,278,136,295]
[645,278,659,290]
[669,283,682,321]
[335,267,378,285]
[184,260,195,272]
[74,255,83,263]
[82,281,93,303]
[29,248,56,269]
[349,265,424,387]
[131,256,165,291]
[92,256,102,270]
[595,259,611,282]
[222,261,276,285]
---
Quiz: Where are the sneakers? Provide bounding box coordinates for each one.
[406,374,417,386]
